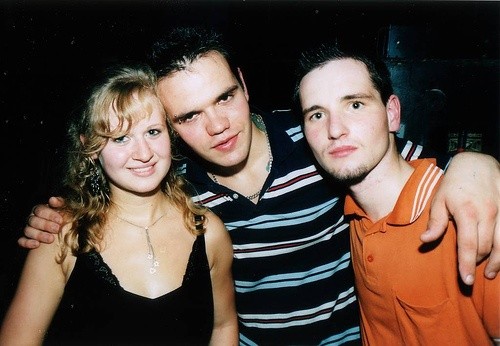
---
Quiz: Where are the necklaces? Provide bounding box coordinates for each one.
[106,205,176,266]
[209,130,274,201]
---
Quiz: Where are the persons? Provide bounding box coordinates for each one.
[288,33,500,346]
[16,26,500,346]
[0,66,240,346]
